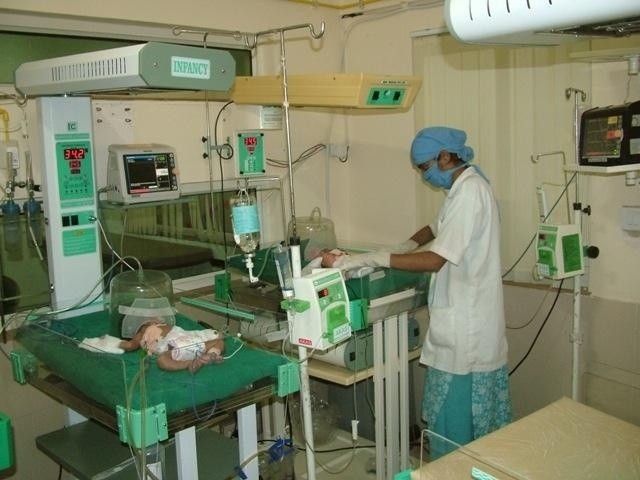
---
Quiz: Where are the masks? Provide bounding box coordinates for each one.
[423,157,460,189]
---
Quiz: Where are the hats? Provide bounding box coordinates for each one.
[412,127,473,165]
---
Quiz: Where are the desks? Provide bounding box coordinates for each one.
[395,397,640,480]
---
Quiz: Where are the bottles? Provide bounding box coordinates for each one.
[139,444,164,480]
[230,195,262,259]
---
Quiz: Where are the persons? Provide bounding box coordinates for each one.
[319,247,375,280]
[118,321,225,374]
[343,125,514,461]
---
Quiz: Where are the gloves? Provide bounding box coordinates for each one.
[340,239,419,271]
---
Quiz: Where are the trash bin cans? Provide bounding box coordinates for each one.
[0,410,17,480]
